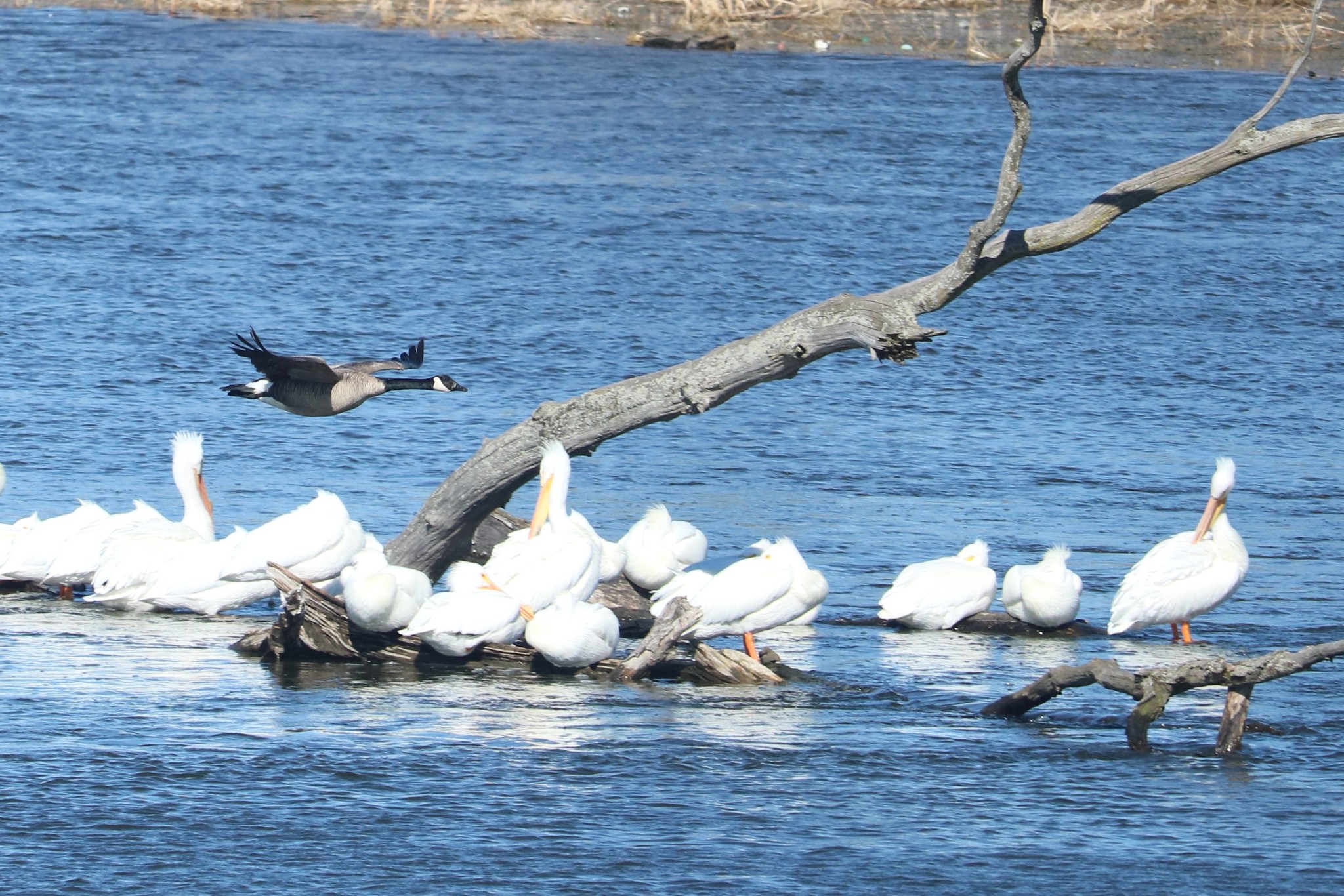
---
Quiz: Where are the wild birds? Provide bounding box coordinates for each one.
[218,325,468,418]
[875,538,998,632]
[1001,541,1084,628]
[0,428,831,670]
[1106,455,1251,644]
